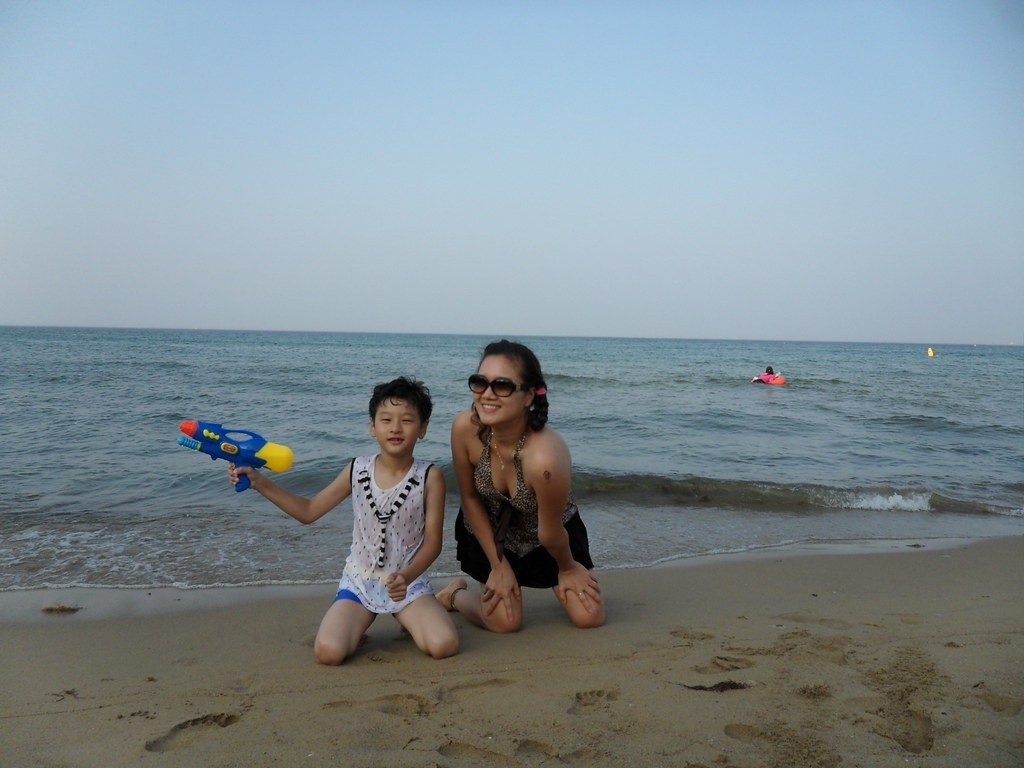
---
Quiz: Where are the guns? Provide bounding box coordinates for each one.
[176,420,295,492]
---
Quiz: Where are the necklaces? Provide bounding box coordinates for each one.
[491,433,515,470]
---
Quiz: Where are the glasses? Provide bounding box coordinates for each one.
[467,373,529,398]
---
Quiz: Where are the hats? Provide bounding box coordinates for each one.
[765,366,773,371]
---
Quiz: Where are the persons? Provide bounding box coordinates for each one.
[432,339,606,633]
[228,377,459,665]
[753,366,779,384]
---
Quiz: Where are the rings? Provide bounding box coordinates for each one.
[578,592,584,596]
